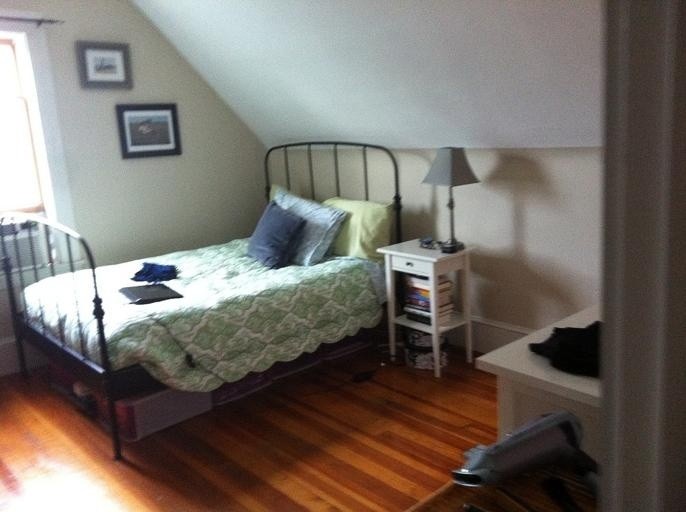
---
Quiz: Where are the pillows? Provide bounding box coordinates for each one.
[244,185,394,270]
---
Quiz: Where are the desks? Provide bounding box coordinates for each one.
[475,305,603,479]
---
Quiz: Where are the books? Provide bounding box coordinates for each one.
[402,271,458,327]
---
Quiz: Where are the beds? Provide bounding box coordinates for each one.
[0,142,401,461]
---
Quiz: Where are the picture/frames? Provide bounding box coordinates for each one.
[75,41,133,89]
[116,104,182,158]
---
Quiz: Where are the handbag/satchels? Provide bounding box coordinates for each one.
[409,335,446,371]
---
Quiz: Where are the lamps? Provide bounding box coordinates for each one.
[421,148,480,251]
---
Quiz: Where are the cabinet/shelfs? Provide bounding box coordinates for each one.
[376,238,473,378]
